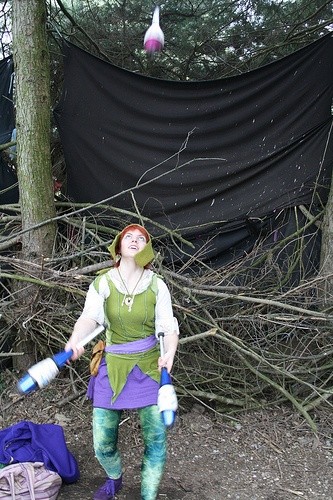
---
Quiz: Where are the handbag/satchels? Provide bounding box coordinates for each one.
[89,340,104,375]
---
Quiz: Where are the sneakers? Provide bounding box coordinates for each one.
[92,476,122,500]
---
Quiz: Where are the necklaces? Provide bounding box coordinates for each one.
[116,267,145,306]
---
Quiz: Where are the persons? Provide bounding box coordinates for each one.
[65,224,180,500]
